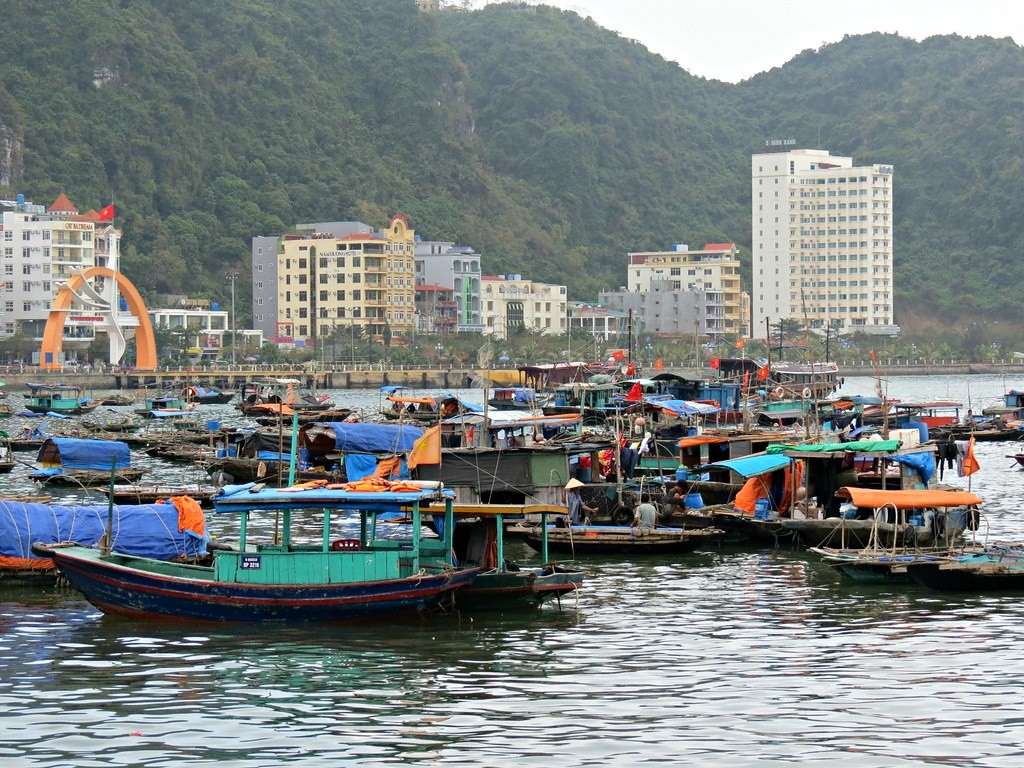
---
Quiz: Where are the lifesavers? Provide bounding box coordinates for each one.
[902,527,918,548]
[967,504,980,531]
[775,387,784,398]
[803,388,811,398]
[612,506,634,526]
[931,510,946,539]
[832,365,837,370]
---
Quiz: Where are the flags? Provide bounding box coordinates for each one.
[408,426,441,469]
[710,357,719,368]
[626,382,641,400]
[758,365,768,380]
[735,340,744,348]
[964,437,980,476]
[613,351,623,360]
[100,205,114,220]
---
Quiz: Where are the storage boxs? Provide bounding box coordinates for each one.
[793,507,824,521]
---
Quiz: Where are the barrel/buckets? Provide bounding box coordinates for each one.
[554,398,565,406]
[674,467,700,481]
[228,445,236,458]
[841,503,859,519]
[908,515,923,525]
[217,450,227,458]
[685,494,705,509]
[688,427,697,437]
[207,421,220,430]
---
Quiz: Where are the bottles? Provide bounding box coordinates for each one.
[754,497,770,519]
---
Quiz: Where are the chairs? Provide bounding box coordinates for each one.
[332,540,361,551]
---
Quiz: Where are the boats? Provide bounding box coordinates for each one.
[378,386,446,420]
[179,385,235,404]
[231,376,336,410]
[33,482,482,623]
[1006,454,1024,468]
[0,437,45,446]
[383,504,583,601]
[805,502,918,581]
[48,422,425,487]
[255,408,357,427]
[94,484,221,505]
[0,430,17,473]
[439,309,909,547]
[0,501,202,585]
[891,553,1024,593]
[135,396,199,418]
[0,381,146,432]
[28,438,148,484]
[896,389,1024,439]
[707,488,984,547]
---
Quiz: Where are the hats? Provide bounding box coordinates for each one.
[564,478,585,488]
[996,414,1000,417]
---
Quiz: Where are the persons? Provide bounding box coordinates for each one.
[663,479,687,515]
[794,487,818,520]
[991,415,1003,430]
[963,410,977,426]
[407,403,415,412]
[630,494,657,530]
[556,478,599,528]
